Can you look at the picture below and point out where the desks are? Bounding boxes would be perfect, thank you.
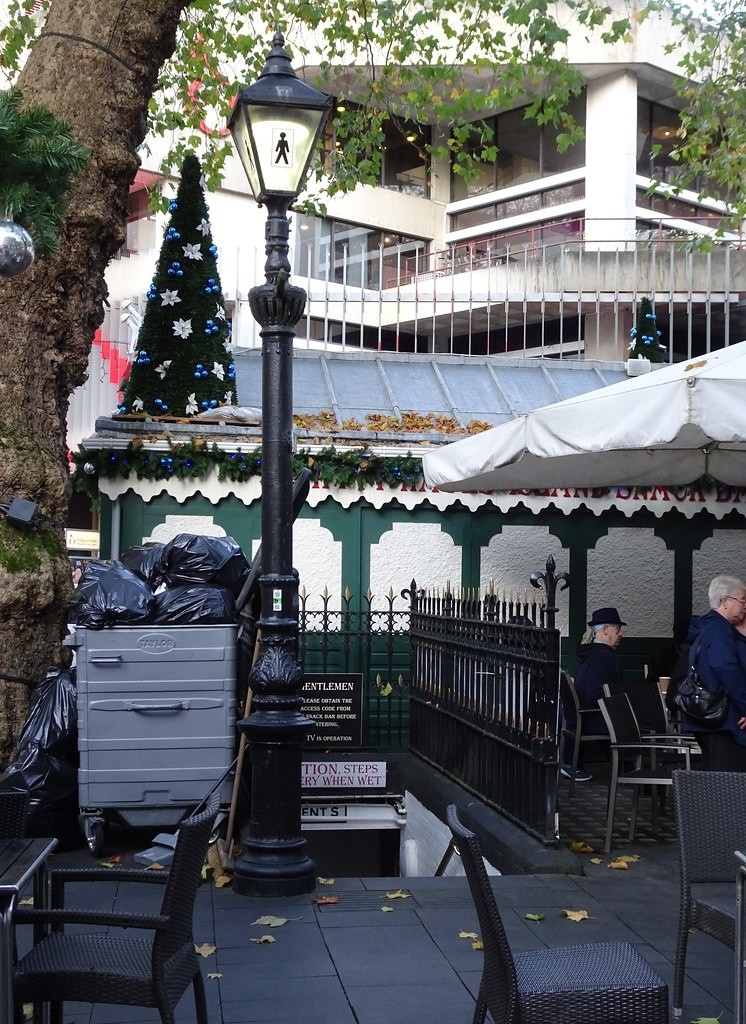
[0,838,61,1024]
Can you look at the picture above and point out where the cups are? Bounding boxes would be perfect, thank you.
[659,676,671,692]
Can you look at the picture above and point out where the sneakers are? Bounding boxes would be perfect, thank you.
[561,764,592,781]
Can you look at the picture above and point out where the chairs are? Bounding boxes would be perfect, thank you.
[672,769,746,1017]
[13,792,221,1024]
[557,668,612,798]
[597,693,692,853]
[446,804,669,1024]
[601,676,702,833]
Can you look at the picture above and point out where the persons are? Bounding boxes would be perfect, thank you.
[685,574,746,772]
[575,607,665,797]
[507,616,593,782]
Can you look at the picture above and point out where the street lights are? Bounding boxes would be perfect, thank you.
[223,30,323,898]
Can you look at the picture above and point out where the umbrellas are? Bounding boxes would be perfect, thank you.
[422,339,746,493]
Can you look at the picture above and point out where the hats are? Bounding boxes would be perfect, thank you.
[587,608,627,627]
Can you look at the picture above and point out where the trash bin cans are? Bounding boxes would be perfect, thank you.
[61,623,240,857]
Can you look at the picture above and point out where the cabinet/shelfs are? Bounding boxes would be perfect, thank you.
[66,623,238,856]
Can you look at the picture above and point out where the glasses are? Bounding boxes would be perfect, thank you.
[725,595,746,606]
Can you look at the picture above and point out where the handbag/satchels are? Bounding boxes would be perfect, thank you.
[672,666,728,727]
